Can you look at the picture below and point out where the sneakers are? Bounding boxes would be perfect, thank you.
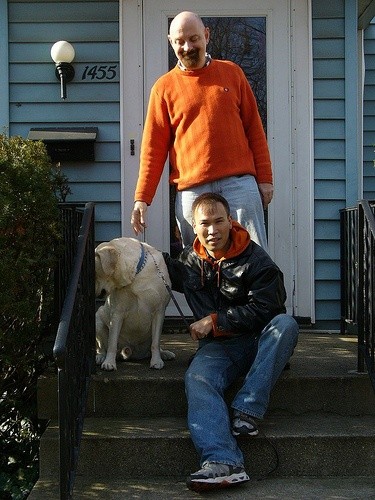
[186,460,249,491]
[232,410,258,436]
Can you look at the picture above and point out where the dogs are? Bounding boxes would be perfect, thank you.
[94,236,176,371]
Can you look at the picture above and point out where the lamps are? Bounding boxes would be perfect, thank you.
[50,40,77,101]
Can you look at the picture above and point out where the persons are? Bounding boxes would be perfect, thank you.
[131,11,274,255]
[157,193,300,494]
